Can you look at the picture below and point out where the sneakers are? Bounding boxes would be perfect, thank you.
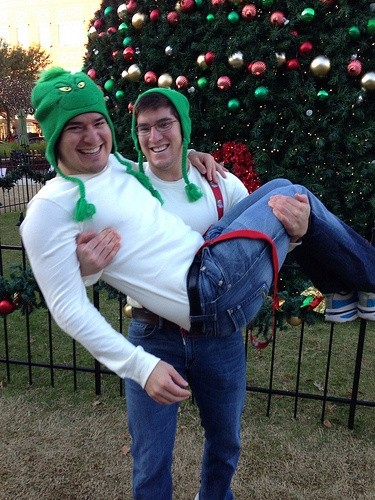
[324,291,374,324]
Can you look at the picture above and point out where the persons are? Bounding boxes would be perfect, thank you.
[76,89,312,500]
[19,66,375,405]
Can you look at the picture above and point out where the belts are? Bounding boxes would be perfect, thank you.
[131,306,175,327]
[187,248,204,335]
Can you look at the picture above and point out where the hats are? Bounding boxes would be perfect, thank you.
[30,67,163,222]
[131,87,202,203]
